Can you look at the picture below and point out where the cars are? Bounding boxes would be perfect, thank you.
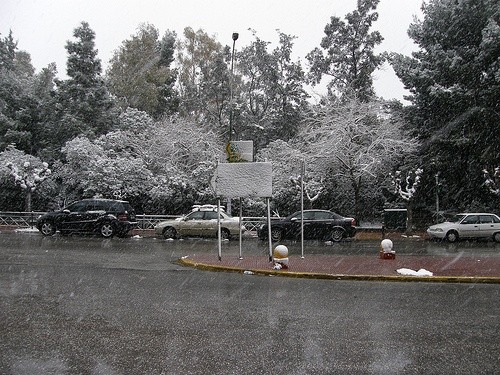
[258,209,359,243]
[154,205,246,241]
[426,213,500,243]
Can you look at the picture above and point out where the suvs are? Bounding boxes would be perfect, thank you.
[36,199,139,239]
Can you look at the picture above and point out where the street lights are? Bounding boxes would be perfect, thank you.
[227,32,240,215]
